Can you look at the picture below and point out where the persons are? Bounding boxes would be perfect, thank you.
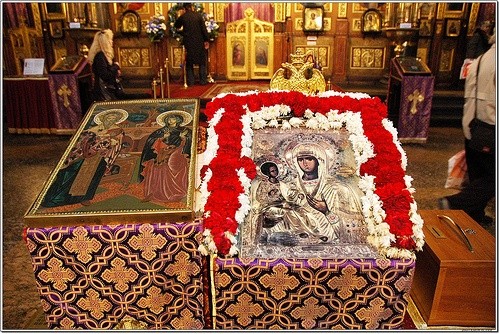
[438,21,496,222]
[173,3,208,85]
[88,28,122,102]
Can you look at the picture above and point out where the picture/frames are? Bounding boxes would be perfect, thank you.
[47,19,65,39]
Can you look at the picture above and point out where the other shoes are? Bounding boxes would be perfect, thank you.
[438,197,452,210]
[479,216,495,225]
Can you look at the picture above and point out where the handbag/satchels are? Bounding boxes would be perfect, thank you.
[444,149,470,190]
[98,77,126,103]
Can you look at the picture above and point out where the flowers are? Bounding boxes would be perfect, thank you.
[145,3,219,42]
